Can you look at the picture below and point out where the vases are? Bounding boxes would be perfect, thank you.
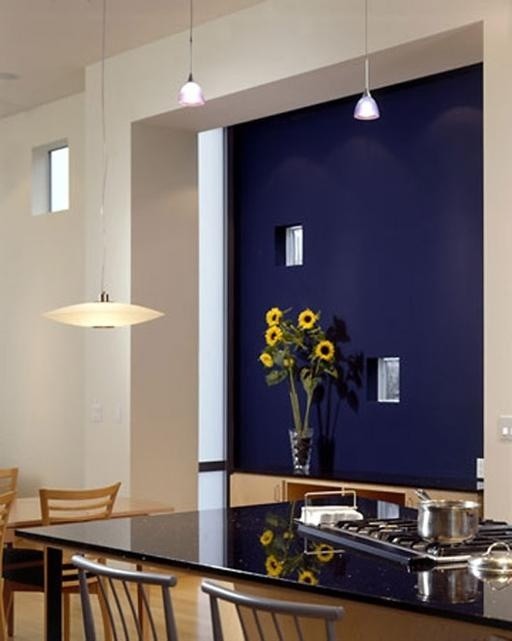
[288,429,317,474]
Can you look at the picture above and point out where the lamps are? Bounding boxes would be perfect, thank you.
[180,1,208,108]
[352,0,382,120]
[42,0,164,332]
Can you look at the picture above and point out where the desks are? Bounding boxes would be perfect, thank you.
[0,495,175,641]
[14,495,512,641]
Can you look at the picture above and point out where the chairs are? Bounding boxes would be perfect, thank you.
[0,466,18,501]
[70,554,184,640]
[200,581,345,641]
[21,483,122,640]
[1,492,22,640]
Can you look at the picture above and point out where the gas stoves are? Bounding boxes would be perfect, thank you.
[295,517,511,565]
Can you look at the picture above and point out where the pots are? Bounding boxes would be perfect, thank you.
[415,569,479,607]
[411,489,481,544]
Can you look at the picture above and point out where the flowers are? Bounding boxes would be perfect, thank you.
[260,502,338,588]
[258,306,342,428]
